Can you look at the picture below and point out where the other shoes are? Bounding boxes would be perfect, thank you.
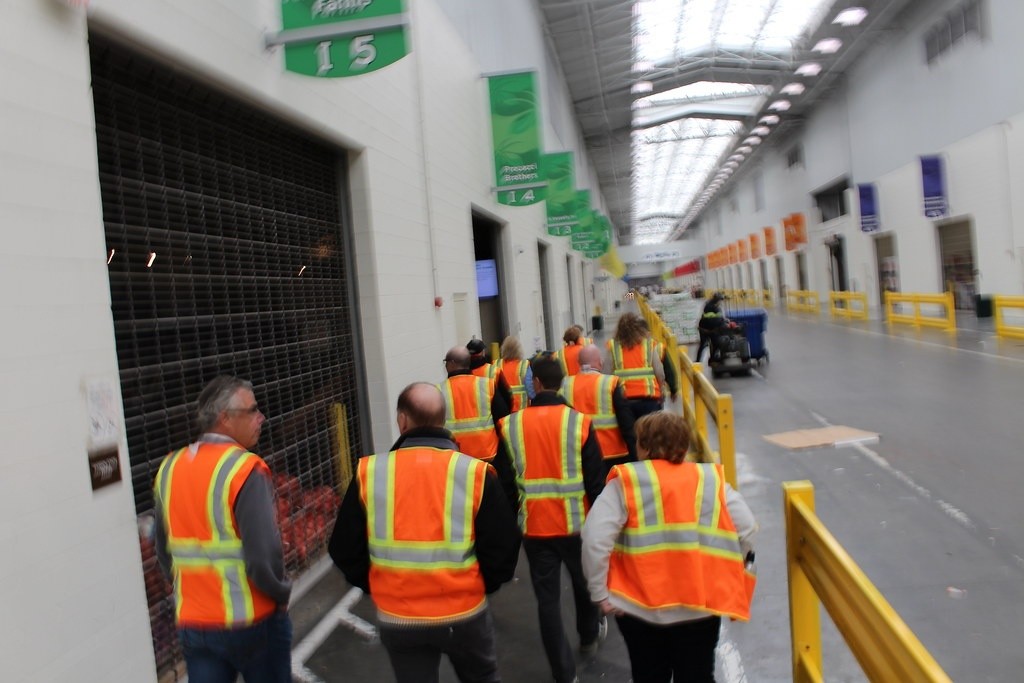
[579,616,611,657]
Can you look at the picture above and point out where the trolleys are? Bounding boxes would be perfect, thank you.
[727,310,769,366]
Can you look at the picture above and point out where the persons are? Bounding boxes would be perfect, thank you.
[490,354,608,683]
[579,409,760,683]
[326,380,524,683]
[148,372,299,683]
[693,291,728,366]
[439,307,678,601]
[636,283,664,302]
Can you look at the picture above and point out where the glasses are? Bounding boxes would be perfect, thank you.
[443,358,452,363]
[225,402,259,415]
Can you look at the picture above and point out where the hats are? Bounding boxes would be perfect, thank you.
[531,352,564,383]
[468,339,487,354]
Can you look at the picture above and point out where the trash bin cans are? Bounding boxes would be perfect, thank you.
[725,306,769,364]
